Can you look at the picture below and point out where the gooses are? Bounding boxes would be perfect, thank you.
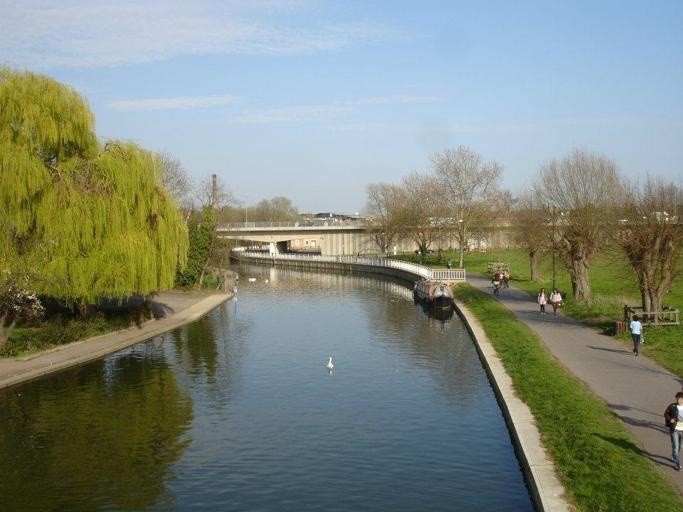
[326,356,335,370]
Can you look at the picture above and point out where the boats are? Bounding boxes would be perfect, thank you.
[414,279,454,308]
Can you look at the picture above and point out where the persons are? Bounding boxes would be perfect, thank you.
[549,288,562,315]
[447,258,452,269]
[664,392,683,471]
[492,270,510,296]
[630,315,642,356]
[537,288,548,316]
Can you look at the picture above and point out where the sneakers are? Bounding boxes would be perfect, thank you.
[675,463,682,470]
[633,349,639,356]
